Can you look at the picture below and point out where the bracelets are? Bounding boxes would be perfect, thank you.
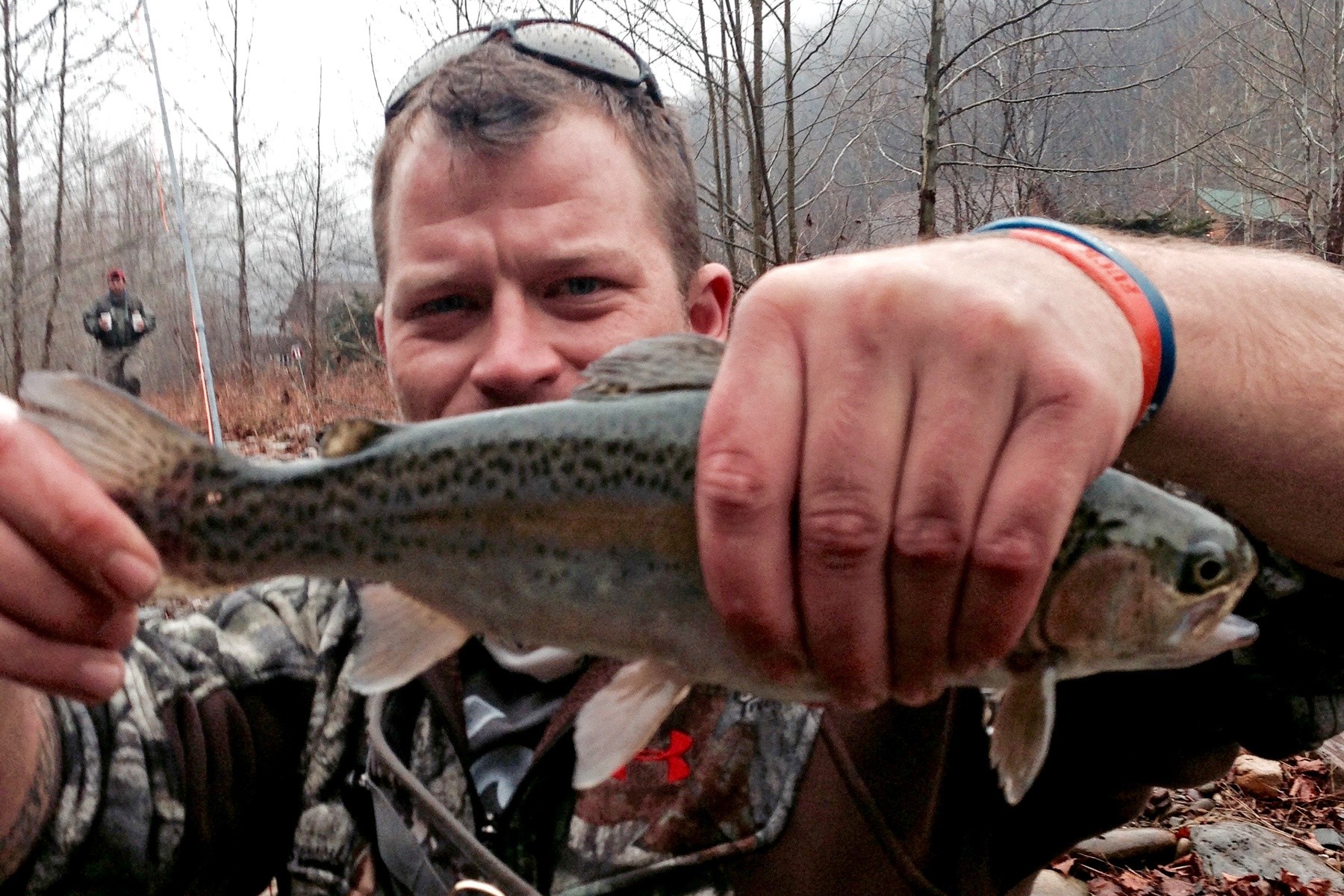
[968,217,1176,439]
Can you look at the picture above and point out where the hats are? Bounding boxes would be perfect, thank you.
[107,268,126,284]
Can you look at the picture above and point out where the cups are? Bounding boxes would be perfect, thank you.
[101,312,112,331]
[131,313,144,332]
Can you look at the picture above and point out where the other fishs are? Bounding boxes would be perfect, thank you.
[13,334,1257,807]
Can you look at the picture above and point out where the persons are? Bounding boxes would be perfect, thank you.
[0,18,1344,896]
[81,269,156,397]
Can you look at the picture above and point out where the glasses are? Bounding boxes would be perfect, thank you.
[384,19,668,128]
